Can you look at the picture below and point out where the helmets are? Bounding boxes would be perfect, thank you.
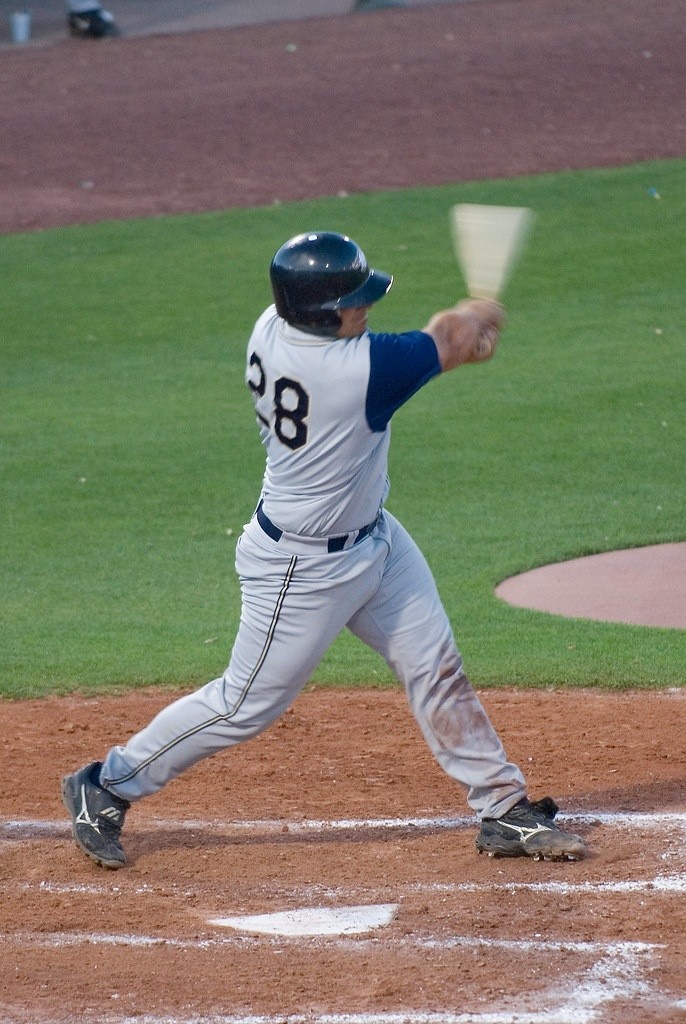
[270,231,394,336]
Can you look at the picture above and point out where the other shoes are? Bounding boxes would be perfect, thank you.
[66,8,117,39]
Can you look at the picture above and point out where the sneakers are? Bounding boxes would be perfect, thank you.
[60,761,132,870]
[475,796,587,861]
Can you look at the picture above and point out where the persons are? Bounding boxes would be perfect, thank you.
[63,231,587,870]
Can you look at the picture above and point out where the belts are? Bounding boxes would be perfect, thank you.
[256,501,379,553]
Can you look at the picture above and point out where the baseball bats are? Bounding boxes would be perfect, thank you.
[446,196,528,359]
